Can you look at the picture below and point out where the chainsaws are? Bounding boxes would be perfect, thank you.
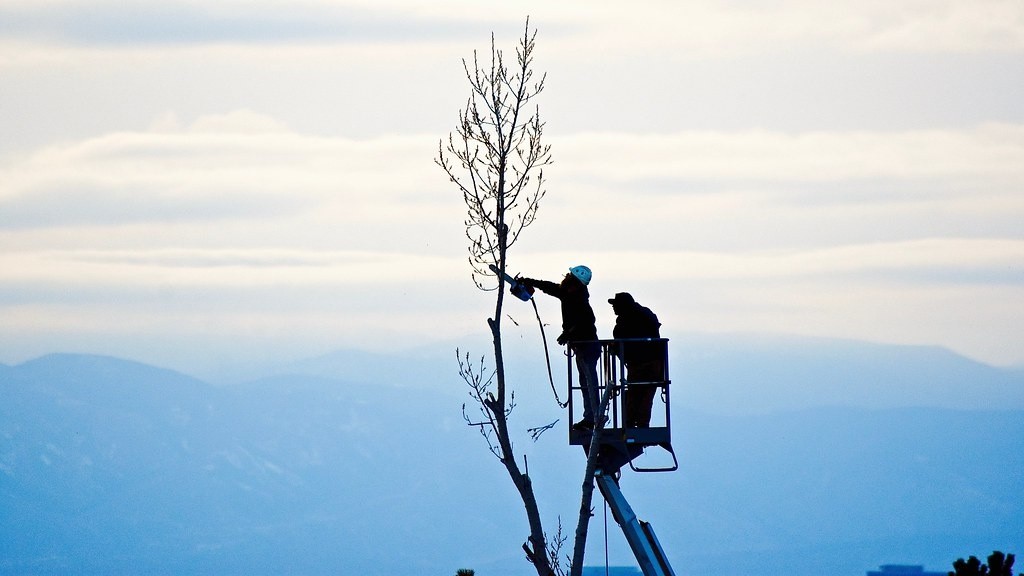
[489,263,535,301]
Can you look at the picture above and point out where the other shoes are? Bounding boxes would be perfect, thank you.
[573,414,606,431]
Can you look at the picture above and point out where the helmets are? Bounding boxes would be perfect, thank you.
[569,265,592,286]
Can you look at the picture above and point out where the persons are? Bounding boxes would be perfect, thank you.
[608,292,661,429]
[525,265,608,432]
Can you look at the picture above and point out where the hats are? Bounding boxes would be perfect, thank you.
[608,292,636,310]
